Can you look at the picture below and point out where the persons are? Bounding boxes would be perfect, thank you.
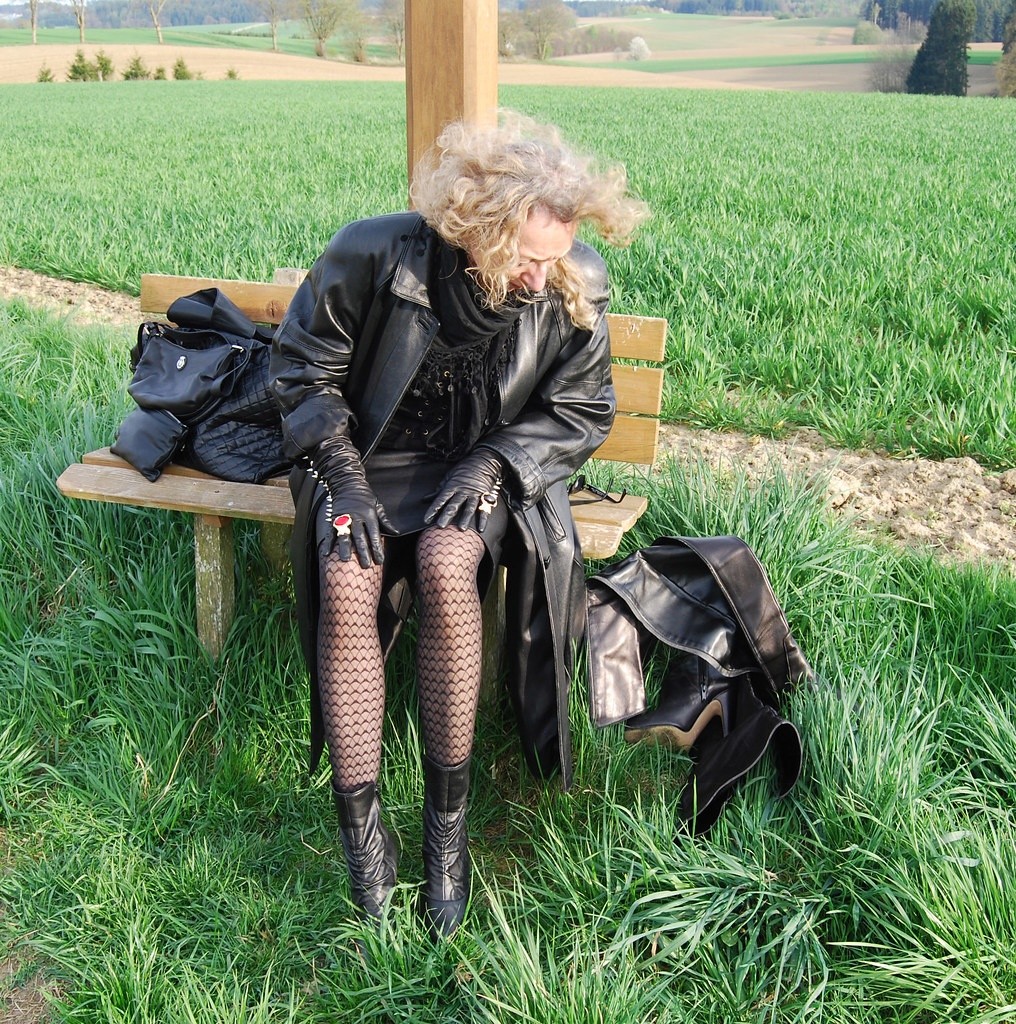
[270,117,648,941]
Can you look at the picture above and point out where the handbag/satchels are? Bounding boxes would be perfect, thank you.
[166,288,292,488]
[110,405,189,482]
[126,321,250,415]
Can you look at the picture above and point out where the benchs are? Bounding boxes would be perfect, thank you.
[54,275,668,664]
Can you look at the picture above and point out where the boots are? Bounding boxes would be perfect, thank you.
[586,536,817,846]
[422,754,470,942]
[333,781,397,969]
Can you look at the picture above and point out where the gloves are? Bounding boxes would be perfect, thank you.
[423,448,507,532]
[302,436,400,569]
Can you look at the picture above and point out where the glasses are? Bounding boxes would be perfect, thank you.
[565,475,627,507]
